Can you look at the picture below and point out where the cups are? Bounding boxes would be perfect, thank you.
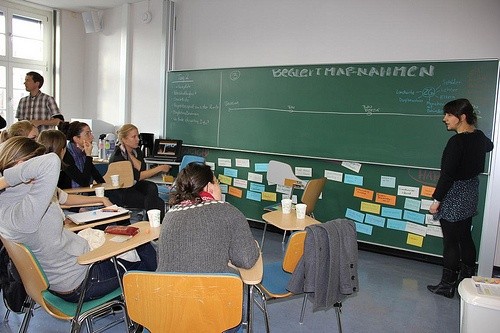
[147,209,161,228]
[110,175,119,187]
[296,203,307,219]
[281,199,292,215]
[94,187,105,197]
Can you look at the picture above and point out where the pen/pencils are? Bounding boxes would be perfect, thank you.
[102,209,118,212]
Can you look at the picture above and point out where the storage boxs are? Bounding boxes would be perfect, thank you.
[458,278,500,333]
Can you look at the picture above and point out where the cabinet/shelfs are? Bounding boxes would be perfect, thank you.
[144,158,180,184]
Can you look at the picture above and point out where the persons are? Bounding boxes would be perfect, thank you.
[426,98,494,297]
[0,71,261,333]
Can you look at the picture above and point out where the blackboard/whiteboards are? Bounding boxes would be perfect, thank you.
[163,58,500,174]
[161,143,490,263]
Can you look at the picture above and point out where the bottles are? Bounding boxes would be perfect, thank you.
[98,134,106,159]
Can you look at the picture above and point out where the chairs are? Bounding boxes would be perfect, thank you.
[0,160,343,332]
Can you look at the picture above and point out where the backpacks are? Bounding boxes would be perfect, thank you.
[0,239,28,314]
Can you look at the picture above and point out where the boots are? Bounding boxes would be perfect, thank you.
[427,267,459,298]
[454,262,474,289]
